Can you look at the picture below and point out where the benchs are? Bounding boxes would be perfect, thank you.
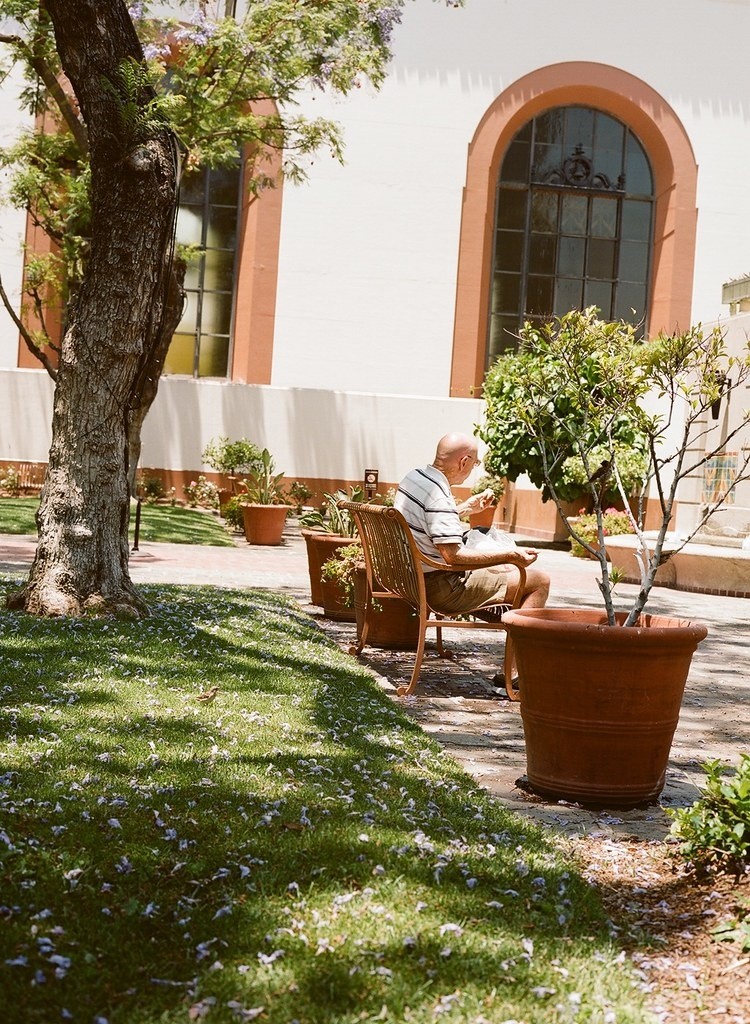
[335,498,526,702]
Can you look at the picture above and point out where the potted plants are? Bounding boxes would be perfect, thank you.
[298,485,394,623]
[200,436,263,518]
[319,538,430,650]
[469,304,750,811]
[469,476,505,529]
[226,449,294,546]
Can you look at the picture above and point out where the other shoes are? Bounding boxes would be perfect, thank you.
[491,670,520,696]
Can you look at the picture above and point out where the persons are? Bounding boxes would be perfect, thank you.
[396,432,550,697]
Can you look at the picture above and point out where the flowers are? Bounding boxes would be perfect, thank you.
[568,508,636,556]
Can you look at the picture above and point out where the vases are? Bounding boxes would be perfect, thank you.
[566,516,590,558]
[588,530,610,562]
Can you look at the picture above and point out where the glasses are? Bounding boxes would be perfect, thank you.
[461,455,481,466]
